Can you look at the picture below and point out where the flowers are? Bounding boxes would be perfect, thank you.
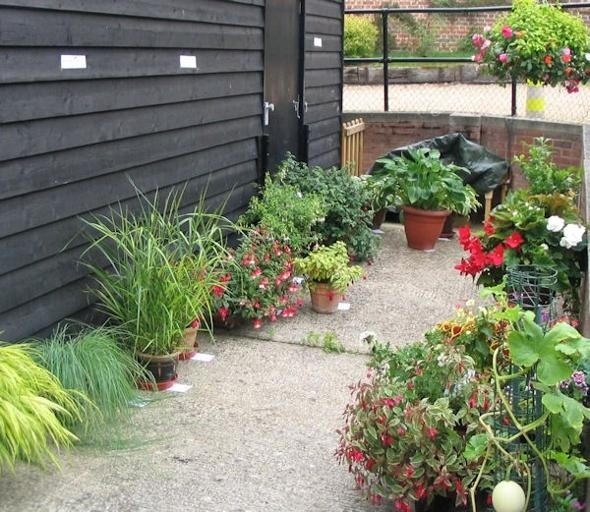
[291,241,367,301]
[455,189,588,287]
[470,1,589,94]
[211,222,304,330]
[334,334,512,512]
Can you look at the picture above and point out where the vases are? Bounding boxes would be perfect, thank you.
[525,77,546,121]
[308,280,344,314]
[415,473,456,511]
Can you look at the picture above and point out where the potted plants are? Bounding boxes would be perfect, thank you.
[357,149,482,249]
